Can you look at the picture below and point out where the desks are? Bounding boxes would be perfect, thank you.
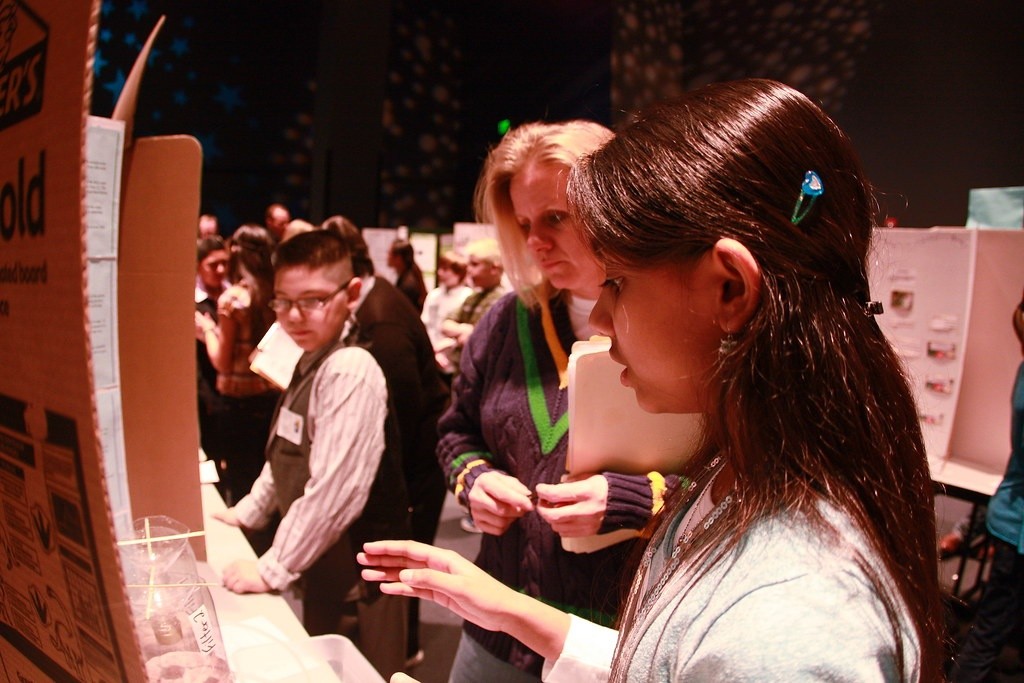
[198,447,336,683]
[926,457,1004,616]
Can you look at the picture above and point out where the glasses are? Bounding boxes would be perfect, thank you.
[267,278,350,313]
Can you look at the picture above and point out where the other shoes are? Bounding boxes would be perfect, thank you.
[935,532,962,556]
[977,544,995,561]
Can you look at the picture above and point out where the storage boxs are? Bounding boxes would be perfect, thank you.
[309,632,386,682]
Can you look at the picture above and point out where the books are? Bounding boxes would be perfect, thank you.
[561,334,702,555]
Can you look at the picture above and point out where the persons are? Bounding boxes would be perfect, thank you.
[195,202,506,506]
[210,231,408,682]
[938,292,1023,683]
[436,120,703,683]
[353,79,961,683]
[341,234,446,668]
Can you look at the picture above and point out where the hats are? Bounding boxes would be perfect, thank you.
[442,251,466,265]
[461,238,504,270]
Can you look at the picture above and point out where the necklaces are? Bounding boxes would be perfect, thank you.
[678,464,726,546]
